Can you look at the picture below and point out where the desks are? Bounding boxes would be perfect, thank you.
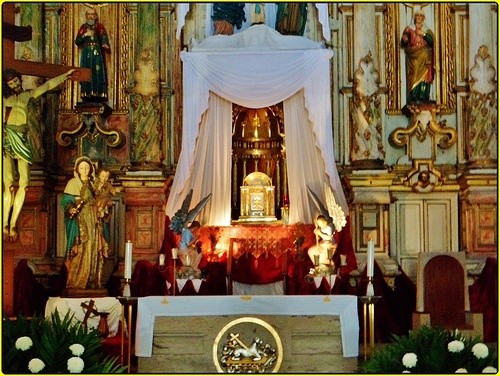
[304,274,341,296]
[45,296,127,338]
[134,295,359,373]
[361,296,382,351]
[176,278,208,296]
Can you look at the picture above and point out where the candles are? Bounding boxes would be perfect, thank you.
[124,240,132,279]
[159,254,165,265]
[227,239,233,272]
[341,254,346,265]
[328,250,332,260]
[367,238,374,277]
[281,238,287,273]
[185,255,191,267]
[314,255,319,265]
[172,248,178,259]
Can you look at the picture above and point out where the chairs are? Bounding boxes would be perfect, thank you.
[412,251,483,341]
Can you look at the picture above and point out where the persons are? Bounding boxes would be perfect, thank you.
[3,69,74,242]
[400,11,437,102]
[178,220,203,273]
[94,168,119,213]
[61,156,113,290]
[75,9,110,104]
[307,215,336,270]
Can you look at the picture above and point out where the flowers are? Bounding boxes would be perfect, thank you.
[2,306,128,374]
[362,324,498,374]
